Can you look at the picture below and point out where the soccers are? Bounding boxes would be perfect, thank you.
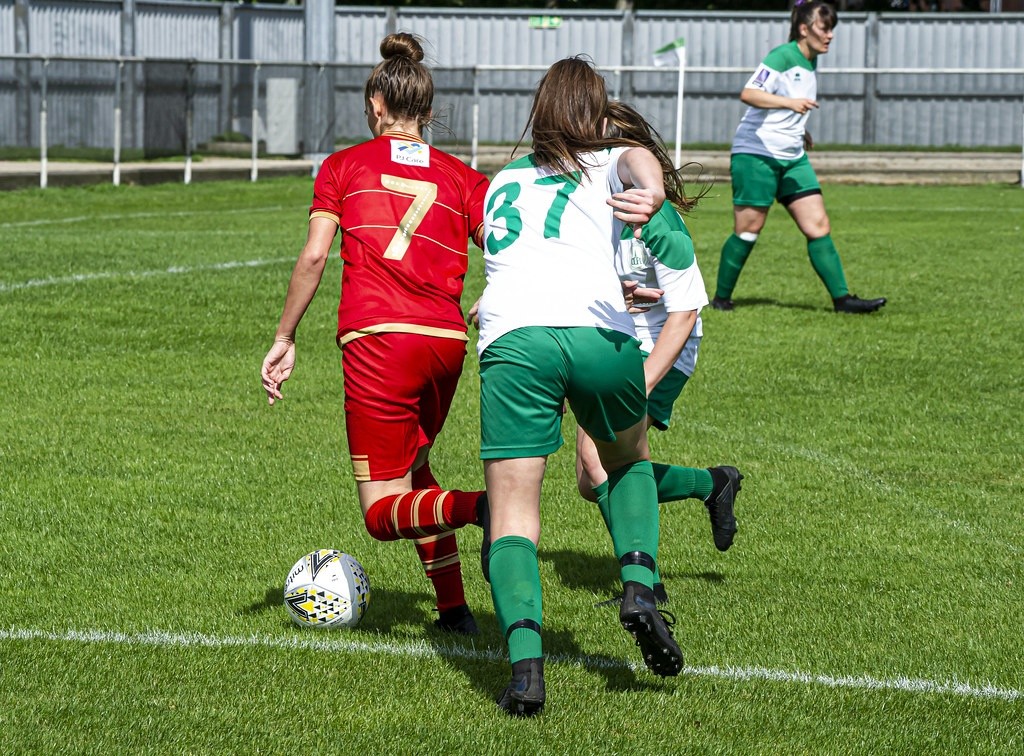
[283,547,372,633]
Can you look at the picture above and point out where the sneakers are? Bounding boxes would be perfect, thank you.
[619,583,685,678]
[482,492,492,584]
[602,583,669,605]
[834,295,888,314]
[496,660,546,718]
[707,466,745,552]
[712,301,736,312]
[431,609,478,635]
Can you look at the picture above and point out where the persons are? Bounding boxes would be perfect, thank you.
[476,53,685,717]
[261,33,492,632]
[709,0,888,312]
[563,103,744,607]
